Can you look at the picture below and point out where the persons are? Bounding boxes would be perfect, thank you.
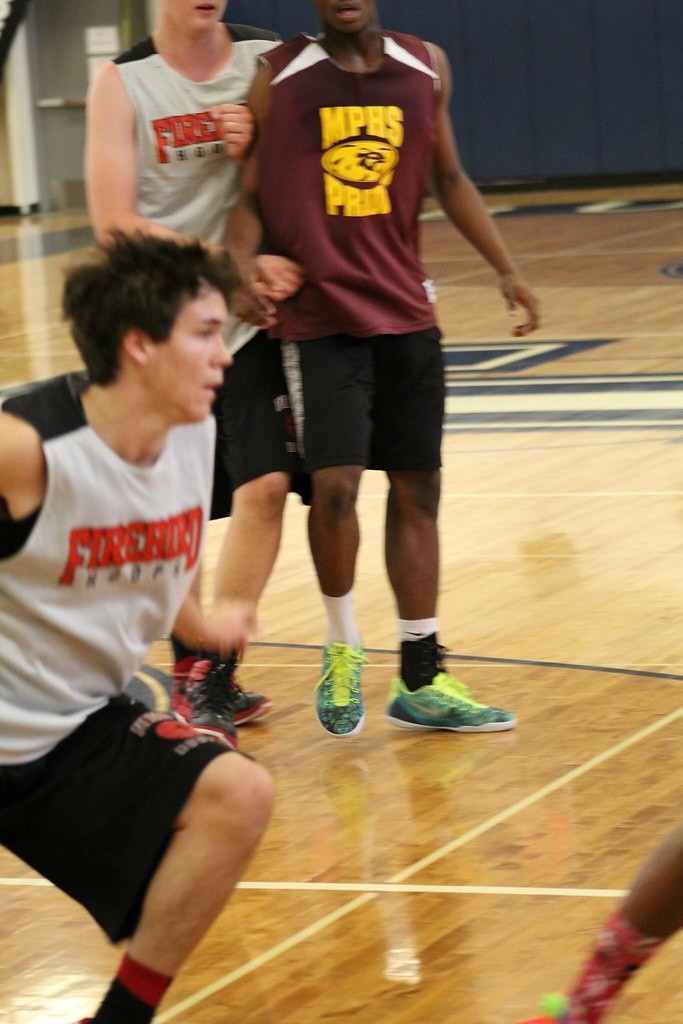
[224,1,541,737]
[0,229,272,1024]
[521,823,683,1024]
[84,1,300,732]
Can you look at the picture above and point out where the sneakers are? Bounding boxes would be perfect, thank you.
[315,634,367,738]
[318,752,378,847]
[387,729,518,793]
[168,635,274,749]
[385,670,518,734]
